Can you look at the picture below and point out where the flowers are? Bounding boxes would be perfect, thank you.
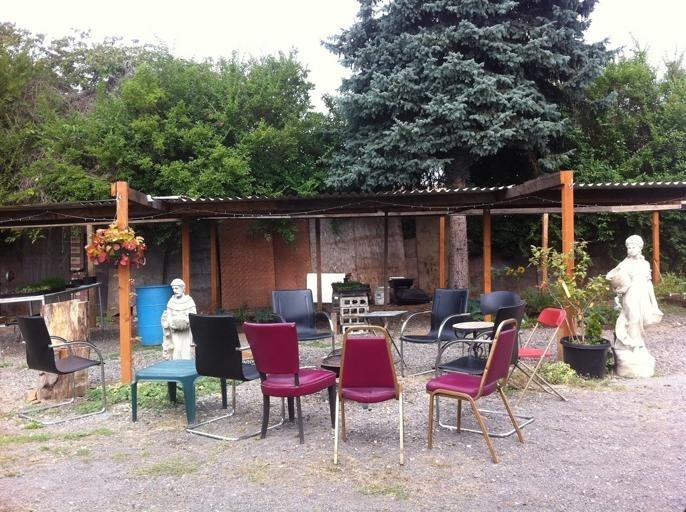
[84,225,149,269]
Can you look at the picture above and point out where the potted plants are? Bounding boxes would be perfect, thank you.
[529,239,612,381]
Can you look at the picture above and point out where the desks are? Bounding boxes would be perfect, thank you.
[451,321,495,362]
[356,311,408,371]
[1,282,103,328]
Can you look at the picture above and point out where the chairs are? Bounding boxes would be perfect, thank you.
[425,317,525,464]
[399,288,470,377]
[271,288,335,355]
[461,290,523,389]
[331,325,406,467]
[436,300,535,437]
[188,312,285,442]
[242,321,336,445]
[16,315,105,427]
[517,306,568,402]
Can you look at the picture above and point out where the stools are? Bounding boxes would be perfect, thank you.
[130,359,227,425]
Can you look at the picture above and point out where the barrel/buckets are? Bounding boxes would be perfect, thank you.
[135,284,174,346]
[374,286,390,305]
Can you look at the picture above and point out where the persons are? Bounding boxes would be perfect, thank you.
[605,234,664,377]
[160,278,198,361]
